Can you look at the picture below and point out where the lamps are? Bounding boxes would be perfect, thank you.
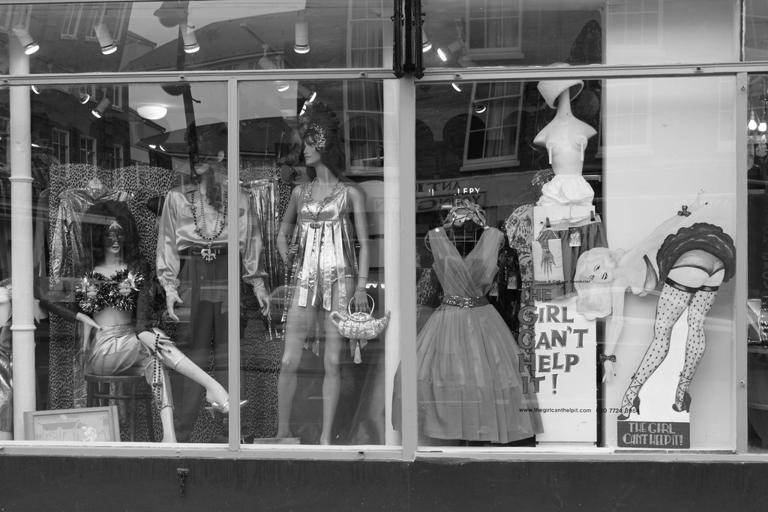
[93,24,118,55]
[11,23,40,55]
[421,29,486,114]
[181,24,200,54]
[31,84,167,120]
[239,22,317,103]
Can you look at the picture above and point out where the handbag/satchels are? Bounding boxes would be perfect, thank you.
[330,294,392,340]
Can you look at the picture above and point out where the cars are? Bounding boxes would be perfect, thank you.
[162,285,247,344]
[530,287,735,363]
[262,285,298,330]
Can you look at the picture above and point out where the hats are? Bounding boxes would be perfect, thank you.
[536,61,583,97]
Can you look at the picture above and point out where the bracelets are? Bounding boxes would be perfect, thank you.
[597,352,616,364]
[674,205,692,218]
[356,275,368,279]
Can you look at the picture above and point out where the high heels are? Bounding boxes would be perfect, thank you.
[205,398,248,418]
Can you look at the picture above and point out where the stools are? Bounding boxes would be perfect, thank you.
[85,371,154,441]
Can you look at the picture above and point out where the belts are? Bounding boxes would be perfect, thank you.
[189,247,226,256]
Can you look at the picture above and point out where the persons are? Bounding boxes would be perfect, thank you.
[154,115,270,440]
[40,199,248,442]
[407,200,544,446]
[573,185,737,422]
[530,79,599,221]
[268,109,371,444]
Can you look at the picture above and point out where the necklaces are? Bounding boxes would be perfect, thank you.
[305,176,339,230]
[190,181,225,240]
